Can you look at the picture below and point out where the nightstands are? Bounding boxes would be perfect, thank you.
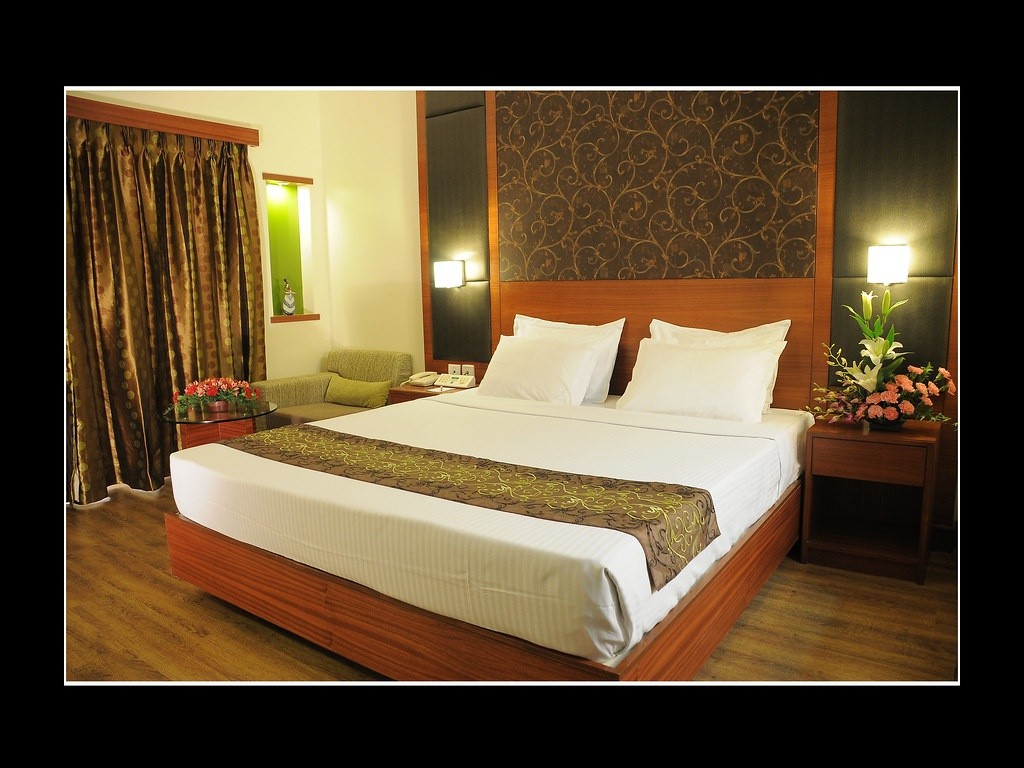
[390,376,480,405]
[799,416,941,584]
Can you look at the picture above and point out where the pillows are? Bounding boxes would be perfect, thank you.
[327,371,391,409]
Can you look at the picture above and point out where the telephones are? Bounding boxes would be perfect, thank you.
[408,371,438,387]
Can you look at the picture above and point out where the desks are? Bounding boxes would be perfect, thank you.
[157,396,277,452]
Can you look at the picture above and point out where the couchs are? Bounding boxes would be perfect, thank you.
[249,347,414,433]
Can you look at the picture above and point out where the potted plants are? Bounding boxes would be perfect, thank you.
[206,398,228,413]
[865,407,907,432]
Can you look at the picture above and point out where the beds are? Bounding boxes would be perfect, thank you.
[162,272,823,679]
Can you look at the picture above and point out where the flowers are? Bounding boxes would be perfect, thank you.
[808,288,957,433]
[169,376,259,413]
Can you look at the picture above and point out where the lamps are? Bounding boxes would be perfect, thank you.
[432,260,468,291]
[267,184,289,206]
[864,239,918,289]
